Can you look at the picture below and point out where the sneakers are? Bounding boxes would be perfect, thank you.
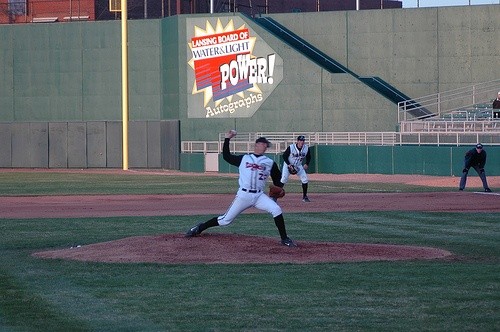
[187,222,205,237]
[281,238,298,247]
[302,197,310,202]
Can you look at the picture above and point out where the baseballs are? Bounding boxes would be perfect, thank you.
[230,129,237,136]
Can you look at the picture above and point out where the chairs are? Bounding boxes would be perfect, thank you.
[440,104,500,128]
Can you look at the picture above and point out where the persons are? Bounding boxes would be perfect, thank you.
[185,129,299,249]
[491,91,500,119]
[459,142,491,192]
[280,136,312,203]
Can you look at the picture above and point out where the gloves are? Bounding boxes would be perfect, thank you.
[480,169,484,172]
[463,168,468,174]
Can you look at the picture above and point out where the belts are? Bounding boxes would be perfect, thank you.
[242,189,260,193]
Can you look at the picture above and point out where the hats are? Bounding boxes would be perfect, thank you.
[297,136,305,141]
[256,137,271,147]
[476,144,483,149]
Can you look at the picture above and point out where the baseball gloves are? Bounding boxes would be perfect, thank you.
[268,184,286,199]
[288,165,299,175]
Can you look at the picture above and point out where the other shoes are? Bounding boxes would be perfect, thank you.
[485,188,491,192]
[459,188,463,191]
